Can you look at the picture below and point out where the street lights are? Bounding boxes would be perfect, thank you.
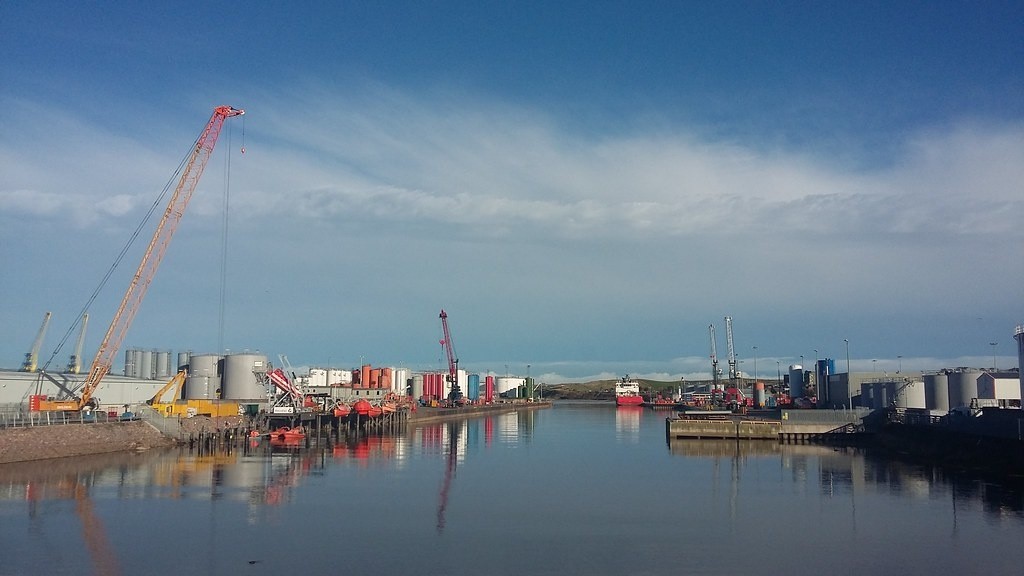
[738,360,745,394]
[800,354,805,383]
[751,346,759,383]
[813,348,820,401]
[990,341,999,374]
[872,359,878,372]
[777,360,781,401]
[897,356,904,378]
[844,338,852,411]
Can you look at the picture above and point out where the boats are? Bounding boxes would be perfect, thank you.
[616,375,644,407]
[250,427,305,449]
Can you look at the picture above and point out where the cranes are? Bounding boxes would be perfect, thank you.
[65,312,89,375]
[22,309,51,373]
[438,307,469,408]
[20,104,245,426]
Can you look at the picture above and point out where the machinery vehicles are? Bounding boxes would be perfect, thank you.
[254,354,417,418]
[141,365,240,422]
